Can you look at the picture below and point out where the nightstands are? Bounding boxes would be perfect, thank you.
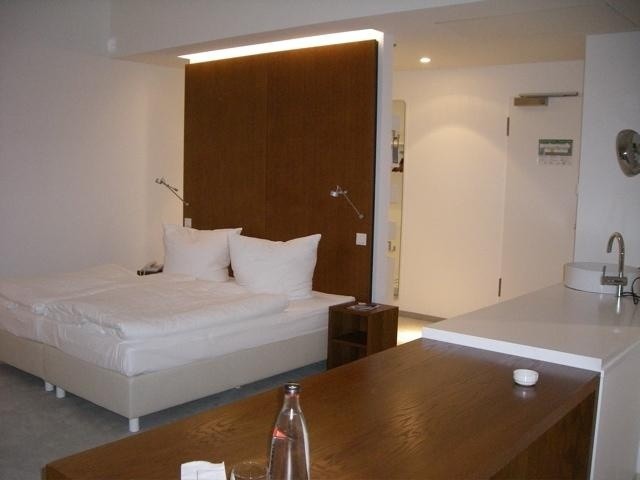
[327,300,399,370]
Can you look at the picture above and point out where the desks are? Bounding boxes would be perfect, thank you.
[41,337,601,480]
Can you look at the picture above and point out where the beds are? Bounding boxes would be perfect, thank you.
[0,265,356,432]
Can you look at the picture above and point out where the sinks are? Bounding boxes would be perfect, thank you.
[563,261,640,295]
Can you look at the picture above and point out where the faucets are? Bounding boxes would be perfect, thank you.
[601,232,627,297]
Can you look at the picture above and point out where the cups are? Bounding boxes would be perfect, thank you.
[229,460,269,479]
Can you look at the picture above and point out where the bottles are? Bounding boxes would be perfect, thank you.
[269,383,310,480]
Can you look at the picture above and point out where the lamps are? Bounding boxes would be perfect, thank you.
[156,178,189,207]
[330,186,364,220]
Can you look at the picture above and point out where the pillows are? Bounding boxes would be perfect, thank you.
[228,234,322,298]
[163,223,243,283]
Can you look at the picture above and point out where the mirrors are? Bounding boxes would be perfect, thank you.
[392,99,406,299]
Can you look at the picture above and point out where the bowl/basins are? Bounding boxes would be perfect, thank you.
[514,367,540,388]
